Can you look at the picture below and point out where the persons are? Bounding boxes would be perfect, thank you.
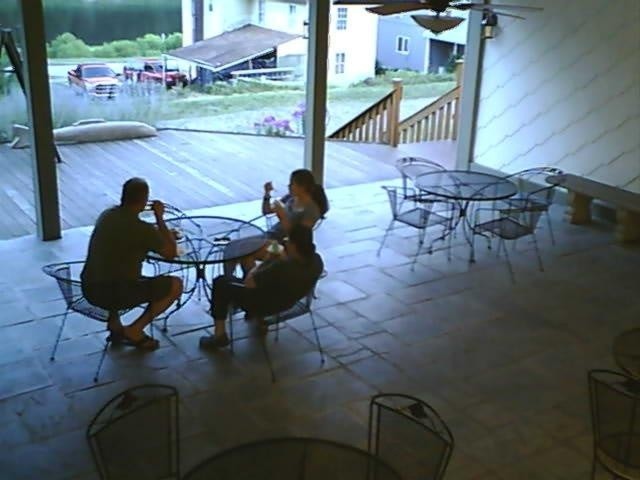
[224,169,329,277]
[200,224,323,349]
[80,176,184,349]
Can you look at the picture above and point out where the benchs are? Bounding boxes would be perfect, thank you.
[545,173,640,243]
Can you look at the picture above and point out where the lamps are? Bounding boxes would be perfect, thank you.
[410,10,465,35]
[482,13,496,38]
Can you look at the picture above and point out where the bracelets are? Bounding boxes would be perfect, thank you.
[264,195,271,201]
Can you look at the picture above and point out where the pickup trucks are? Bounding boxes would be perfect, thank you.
[65,61,124,102]
[123,58,186,90]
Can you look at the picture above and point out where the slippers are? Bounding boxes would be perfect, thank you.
[120,335,160,351]
[105,331,122,342]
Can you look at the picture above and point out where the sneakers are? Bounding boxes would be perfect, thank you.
[199,333,229,349]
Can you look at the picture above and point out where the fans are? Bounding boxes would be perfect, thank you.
[332,0,544,21]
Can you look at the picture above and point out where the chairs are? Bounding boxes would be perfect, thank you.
[478,166,563,247]
[587,368,640,480]
[138,200,203,320]
[86,384,180,480]
[376,186,454,271]
[205,208,281,288]
[470,182,560,281]
[223,271,325,382]
[41,260,177,382]
[395,156,463,245]
[367,393,454,480]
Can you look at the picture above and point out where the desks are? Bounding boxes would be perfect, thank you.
[613,327,640,384]
